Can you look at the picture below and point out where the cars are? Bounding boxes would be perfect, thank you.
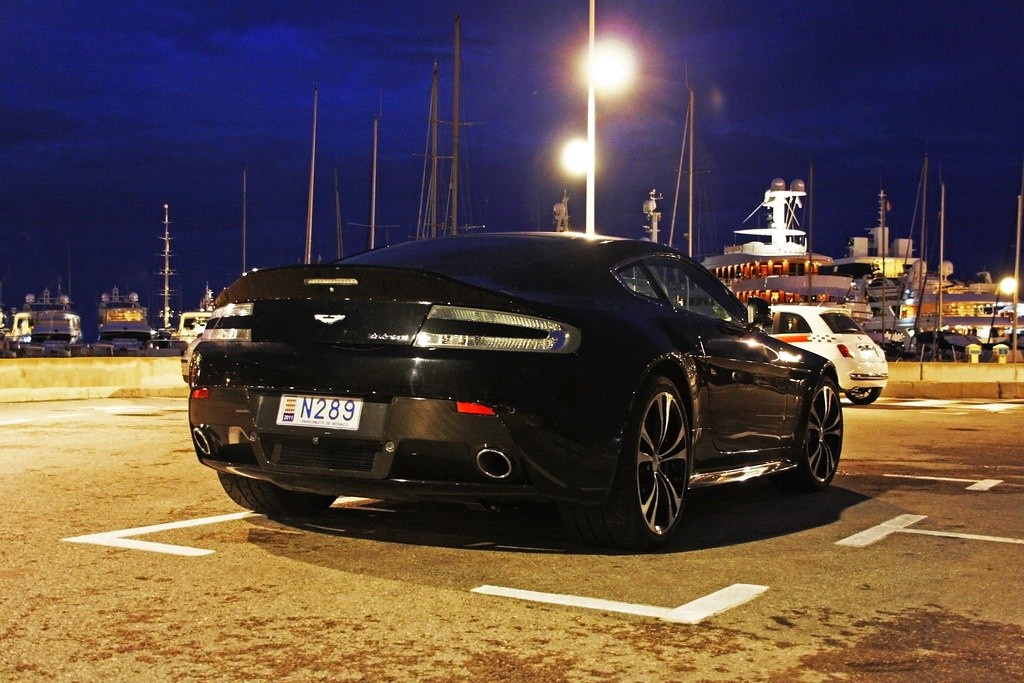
[718,304,888,404]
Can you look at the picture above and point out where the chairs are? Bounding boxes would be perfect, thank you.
[797,317,812,333]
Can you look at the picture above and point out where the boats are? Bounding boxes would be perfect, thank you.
[172,281,221,349]
[699,161,874,348]
[829,155,1023,363]
[3,288,84,355]
[95,286,154,349]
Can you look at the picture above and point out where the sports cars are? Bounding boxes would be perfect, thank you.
[186,229,843,547]
